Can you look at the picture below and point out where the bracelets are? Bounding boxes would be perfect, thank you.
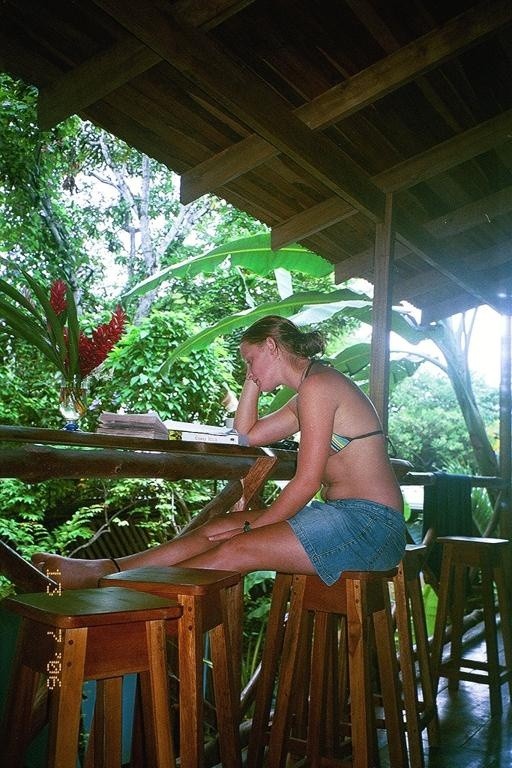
[242,520,253,532]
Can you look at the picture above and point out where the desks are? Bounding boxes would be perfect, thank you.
[1,425,412,482]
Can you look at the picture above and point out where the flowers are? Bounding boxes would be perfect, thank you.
[0,270,129,412]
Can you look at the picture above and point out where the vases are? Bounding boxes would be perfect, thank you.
[58,379,87,431]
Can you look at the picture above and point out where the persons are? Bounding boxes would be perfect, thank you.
[30,314,407,589]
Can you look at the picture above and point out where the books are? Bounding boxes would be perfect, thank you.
[181,432,250,447]
[163,419,238,436]
[95,409,170,440]
